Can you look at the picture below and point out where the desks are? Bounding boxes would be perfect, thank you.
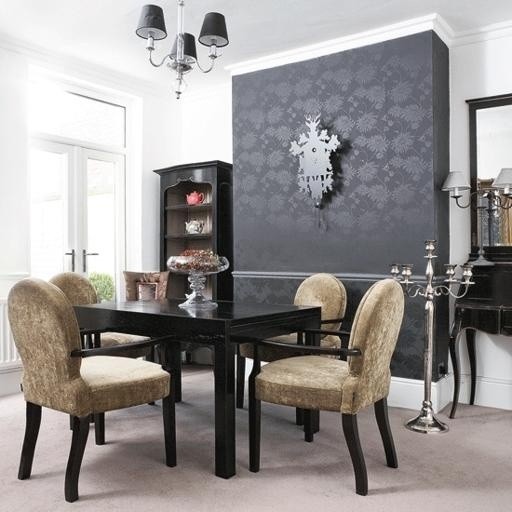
[450,302,512,421]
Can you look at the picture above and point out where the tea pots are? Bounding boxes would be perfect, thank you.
[185,220,205,234]
[186,190,204,206]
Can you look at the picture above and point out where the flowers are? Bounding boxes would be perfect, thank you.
[176,248,217,272]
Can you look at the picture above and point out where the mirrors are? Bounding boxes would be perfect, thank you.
[466,94,512,255]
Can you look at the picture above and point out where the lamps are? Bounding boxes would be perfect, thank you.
[489,165,512,208]
[136,0,229,100]
[439,172,481,208]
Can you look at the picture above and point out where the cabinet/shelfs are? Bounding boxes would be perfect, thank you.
[152,160,234,306]
